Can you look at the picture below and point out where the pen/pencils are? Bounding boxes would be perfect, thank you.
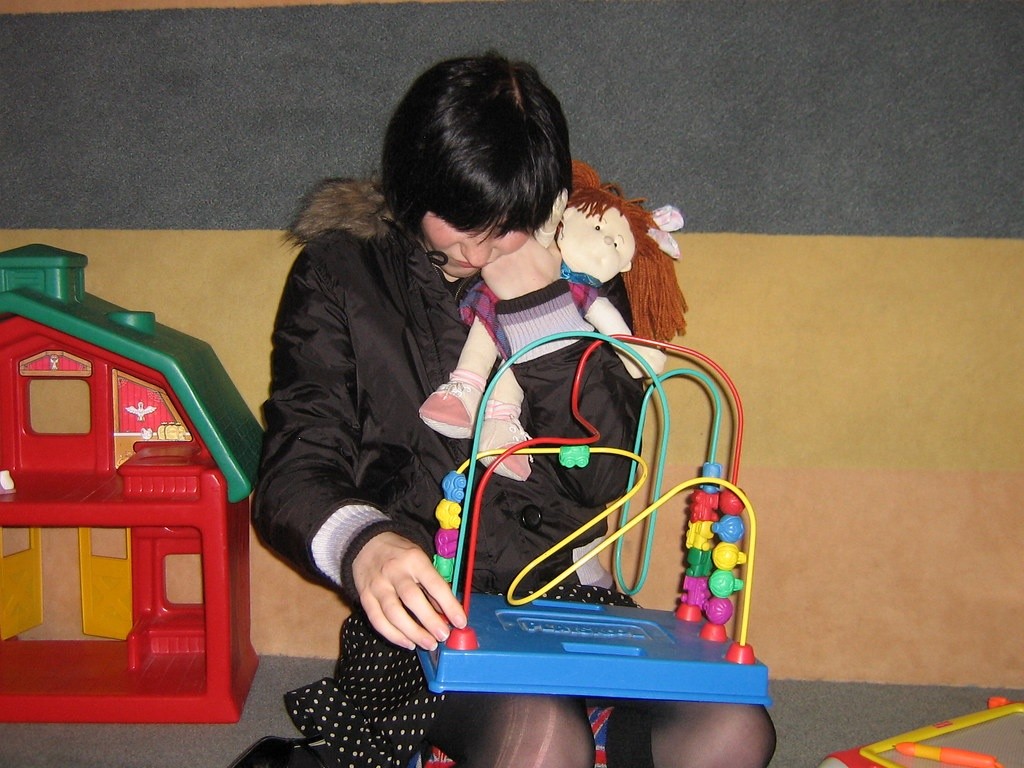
[892,741,1005,768]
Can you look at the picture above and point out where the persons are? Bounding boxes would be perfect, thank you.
[419,160,688,481]
[251,47,777,768]
